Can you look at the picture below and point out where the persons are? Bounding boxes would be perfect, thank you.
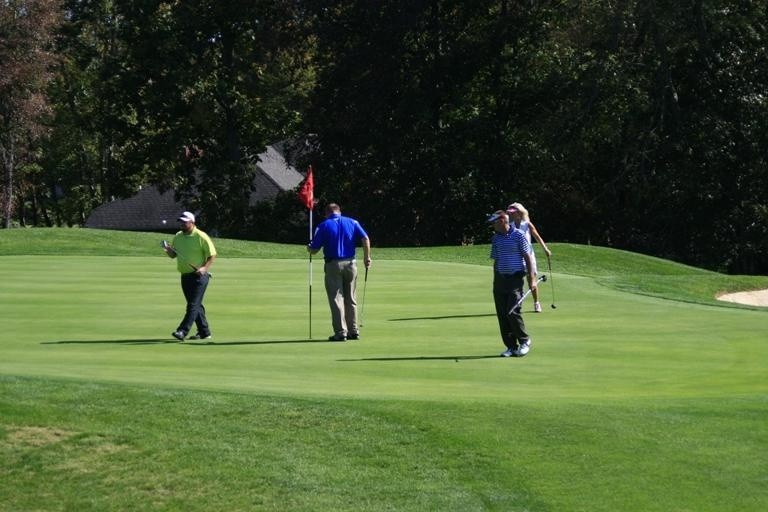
[505,201,553,314]
[307,202,372,342]
[163,210,218,343]
[487,209,538,359]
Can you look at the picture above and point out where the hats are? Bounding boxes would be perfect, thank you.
[487,209,510,224]
[506,207,518,214]
[176,210,195,224]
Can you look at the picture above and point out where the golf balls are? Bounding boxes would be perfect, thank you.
[162,220,167,224]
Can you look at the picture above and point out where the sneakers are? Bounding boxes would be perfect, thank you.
[533,301,542,313]
[328,333,348,343]
[500,346,519,357]
[171,330,187,342]
[189,332,212,340]
[347,332,361,341]
[516,336,532,357]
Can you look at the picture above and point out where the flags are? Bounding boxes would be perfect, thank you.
[296,166,316,210]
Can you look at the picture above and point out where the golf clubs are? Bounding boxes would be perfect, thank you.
[508,275,547,316]
[161,241,198,271]
[360,261,370,327]
[547,254,556,308]
